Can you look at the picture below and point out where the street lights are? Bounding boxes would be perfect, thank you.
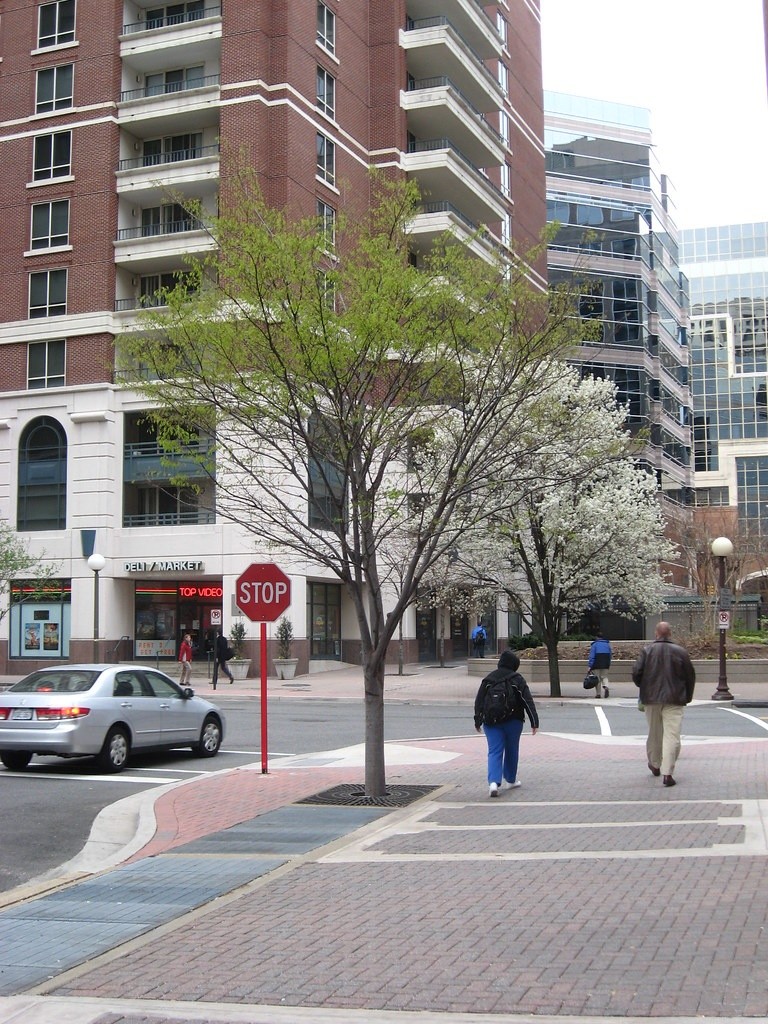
[87,553,106,665]
[711,536,732,701]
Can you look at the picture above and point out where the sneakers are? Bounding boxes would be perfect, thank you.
[489,782,498,797]
[506,781,522,790]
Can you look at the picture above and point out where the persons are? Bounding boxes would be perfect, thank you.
[587,631,612,698]
[472,652,541,799]
[632,621,696,785]
[208,629,235,685]
[471,621,488,658]
[178,633,193,686]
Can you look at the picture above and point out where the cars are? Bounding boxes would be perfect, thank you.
[0,662,228,774]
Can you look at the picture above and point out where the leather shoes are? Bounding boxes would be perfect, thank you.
[647,762,660,776]
[663,775,676,785]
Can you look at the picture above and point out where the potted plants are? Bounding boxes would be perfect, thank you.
[220,611,254,681]
[272,615,299,681]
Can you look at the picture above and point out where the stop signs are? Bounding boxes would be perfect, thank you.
[236,563,291,623]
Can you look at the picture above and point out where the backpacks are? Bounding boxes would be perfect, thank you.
[482,671,521,726]
[476,628,485,646]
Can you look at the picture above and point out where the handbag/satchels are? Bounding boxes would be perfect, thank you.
[225,647,233,661]
[583,668,598,690]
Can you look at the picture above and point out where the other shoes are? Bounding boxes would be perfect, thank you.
[595,695,601,698]
[209,682,217,684]
[605,689,609,698]
[230,677,234,684]
